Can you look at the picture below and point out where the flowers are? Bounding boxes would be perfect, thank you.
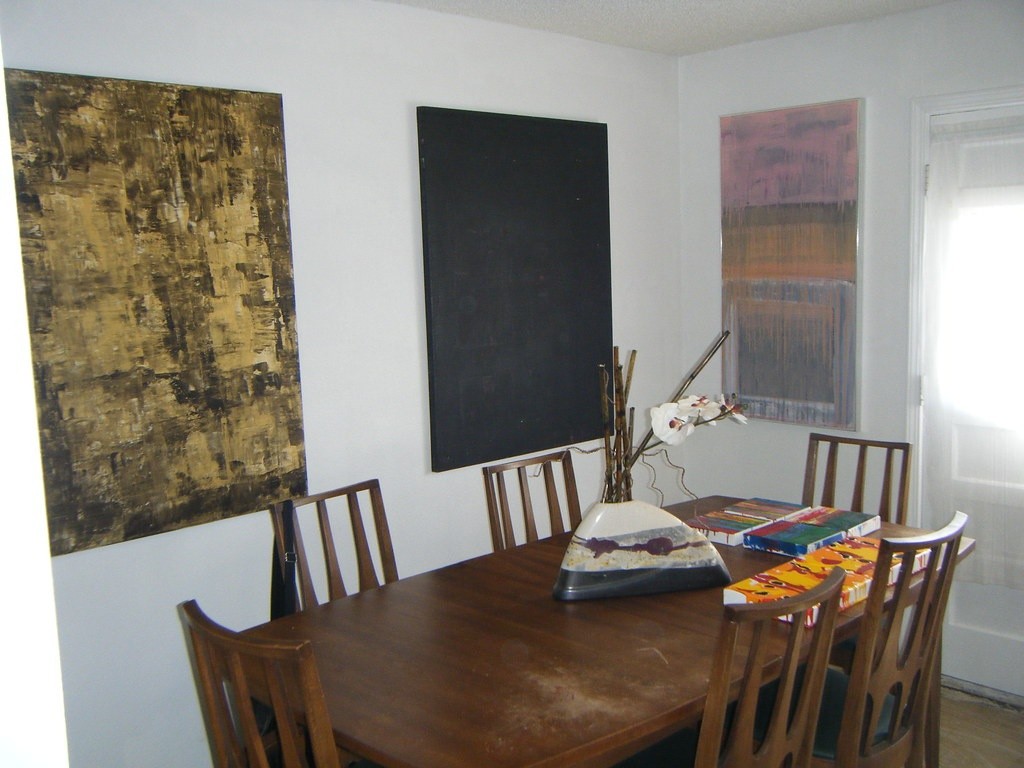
[529,395,745,531]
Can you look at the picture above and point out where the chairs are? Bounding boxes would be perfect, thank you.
[617,511,968,768]
[270,477,401,615]
[176,599,372,768]
[803,432,913,527]
[484,450,583,552]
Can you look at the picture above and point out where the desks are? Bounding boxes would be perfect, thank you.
[213,495,978,768]
